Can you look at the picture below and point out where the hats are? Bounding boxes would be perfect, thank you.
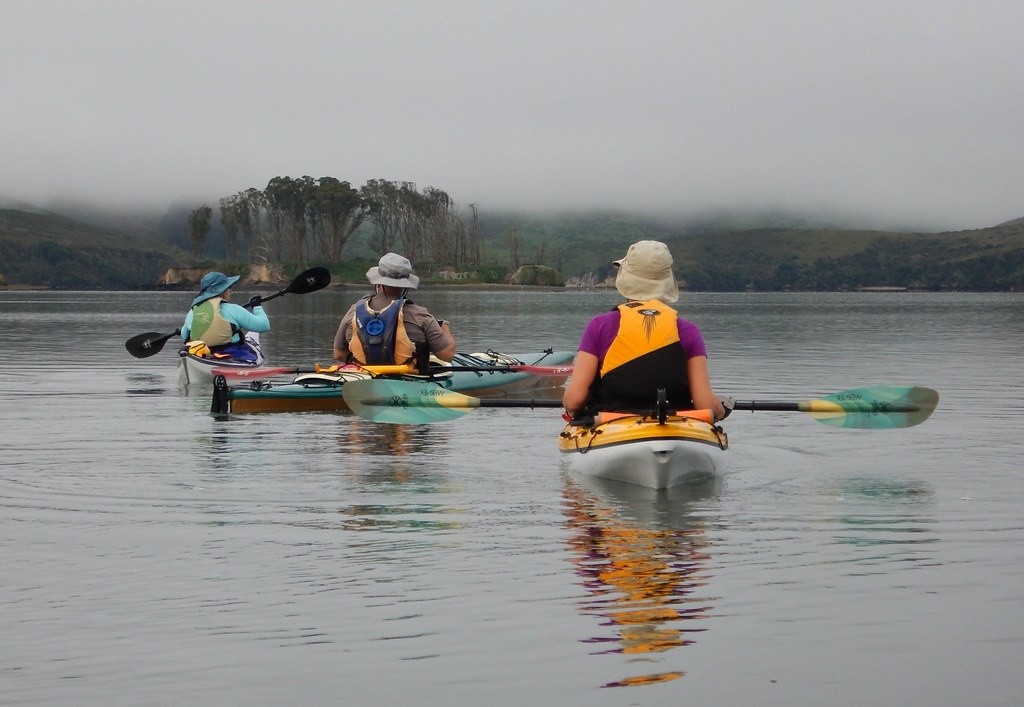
[612,238,680,304]
[191,270,239,309]
[366,252,420,289]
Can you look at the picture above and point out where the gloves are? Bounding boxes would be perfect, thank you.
[713,394,738,421]
[249,295,262,308]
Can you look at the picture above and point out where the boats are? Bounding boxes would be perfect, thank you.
[560,414,730,496]
[178,340,266,386]
[209,346,575,415]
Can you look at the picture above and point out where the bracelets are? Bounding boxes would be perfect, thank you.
[561,409,574,423]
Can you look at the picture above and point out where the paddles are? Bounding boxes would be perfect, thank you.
[337,378,942,432]
[125,266,336,359]
[212,363,577,378]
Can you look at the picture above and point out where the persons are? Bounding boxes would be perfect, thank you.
[563,240,726,423]
[181,272,270,362]
[333,253,456,379]
